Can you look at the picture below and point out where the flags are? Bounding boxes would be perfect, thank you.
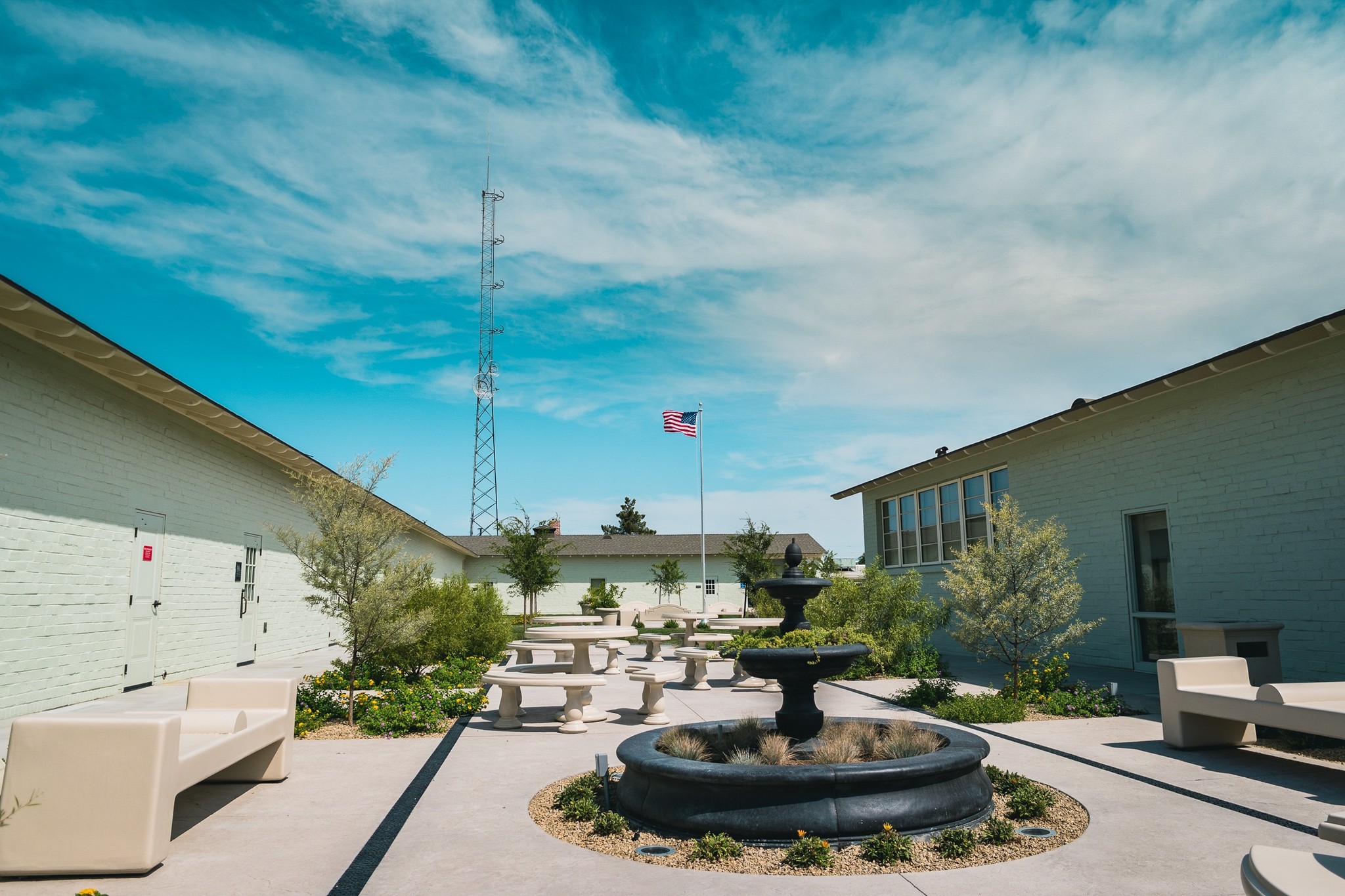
[662,410,699,437]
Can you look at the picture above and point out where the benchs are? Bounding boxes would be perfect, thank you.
[1156,655,1345,750]
[625,663,682,725]
[0,677,298,879]
[674,646,720,690]
[481,662,606,734]
[595,639,631,674]
[506,639,574,664]
[637,633,671,662]
[671,630,733,647]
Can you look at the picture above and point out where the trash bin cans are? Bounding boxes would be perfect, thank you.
[1180,620,1285,687]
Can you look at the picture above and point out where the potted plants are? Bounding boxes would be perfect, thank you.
[578,582,627,625]
[612,715,995,849]
[720,626,871,684]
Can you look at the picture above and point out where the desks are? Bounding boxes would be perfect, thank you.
[533,616,603,644]
[706,618,785,687]
[524,626,639,673]
[661,614,718,660]
